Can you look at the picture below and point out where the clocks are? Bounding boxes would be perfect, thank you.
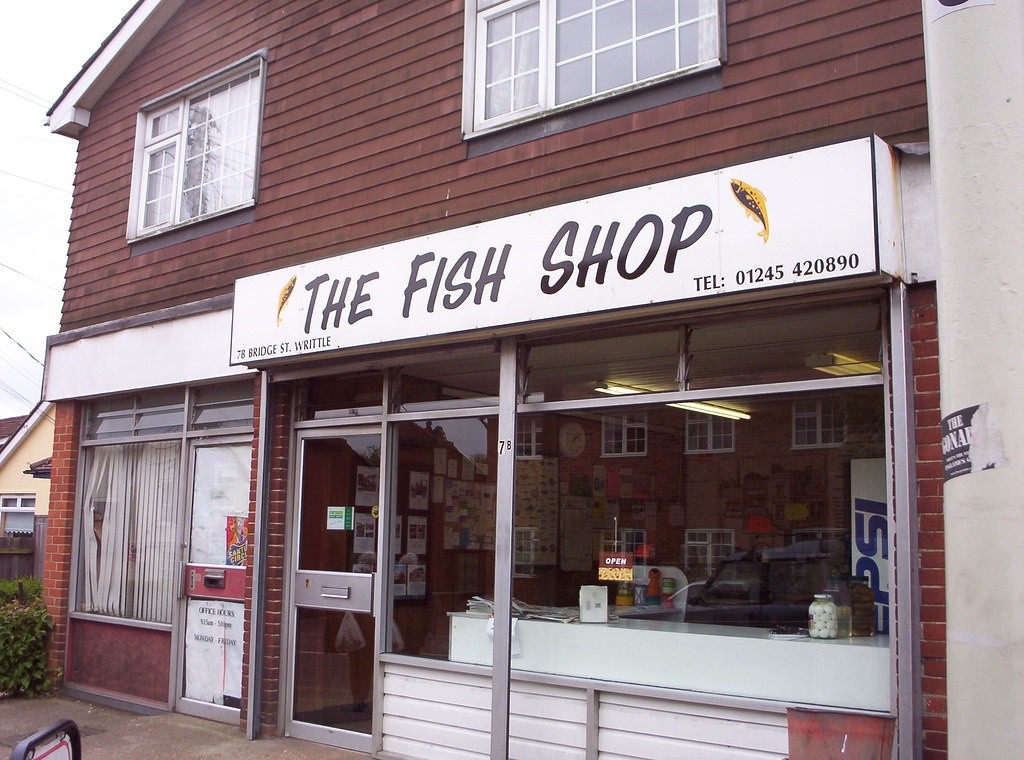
[558,422,585,457]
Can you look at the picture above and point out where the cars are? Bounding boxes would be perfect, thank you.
[655,533,853,631]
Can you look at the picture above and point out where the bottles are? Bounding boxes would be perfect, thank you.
[847,583,876,637]
[822,569,852,637]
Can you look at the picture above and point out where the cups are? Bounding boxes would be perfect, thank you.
[662,578,675,610]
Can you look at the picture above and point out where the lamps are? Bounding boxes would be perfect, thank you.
[594,380,753,421]
[804,352,880,376]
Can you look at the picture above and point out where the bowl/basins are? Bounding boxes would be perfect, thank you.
[818,538,848,553]
